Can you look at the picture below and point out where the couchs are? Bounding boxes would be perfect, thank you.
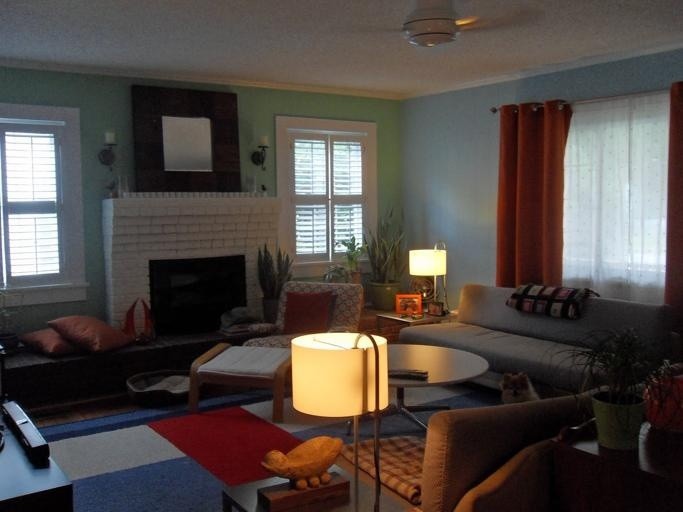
[398,283,671,395]
[334,384,614,512]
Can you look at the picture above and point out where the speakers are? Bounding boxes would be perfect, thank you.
[2,400,50,460]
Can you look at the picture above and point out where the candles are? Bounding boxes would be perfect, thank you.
[259,135,267,145]
[105,128,116,143]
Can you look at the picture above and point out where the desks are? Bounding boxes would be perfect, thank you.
[346,342,491,434]
[0,411,78,512]
[547,430,683,512]
[222,462,405,512]
[375,311,456,336]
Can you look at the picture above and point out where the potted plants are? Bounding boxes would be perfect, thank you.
[257,242,293,324]
[322,236,364,284]
[363,208,406,310]
[537,325,676,453]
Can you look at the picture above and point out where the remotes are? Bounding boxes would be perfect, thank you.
[390,369,428,380]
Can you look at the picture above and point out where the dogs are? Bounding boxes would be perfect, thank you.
[499,370,540,404]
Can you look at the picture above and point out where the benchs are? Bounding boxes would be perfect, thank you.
[2,326,273,407]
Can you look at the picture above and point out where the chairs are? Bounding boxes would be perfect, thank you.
[243,281,364,347]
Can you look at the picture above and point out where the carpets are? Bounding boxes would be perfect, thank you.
[145,403,305,489]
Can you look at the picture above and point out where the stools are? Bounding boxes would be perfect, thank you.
[187,342,294,423]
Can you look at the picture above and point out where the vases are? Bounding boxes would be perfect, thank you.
[642,363,683,435]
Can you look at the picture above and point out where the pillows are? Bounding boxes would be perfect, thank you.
[17,328,81,358]
[282,290,333,334]
[47,314,136,352]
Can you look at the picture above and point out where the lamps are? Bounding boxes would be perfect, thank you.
[407,240,447,318]
[291,332,390,512]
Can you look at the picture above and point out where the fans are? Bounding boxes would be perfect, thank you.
[328,1,540,46]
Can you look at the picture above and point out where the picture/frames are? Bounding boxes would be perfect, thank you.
[395,293,422,317]
[427,301,444,316]
